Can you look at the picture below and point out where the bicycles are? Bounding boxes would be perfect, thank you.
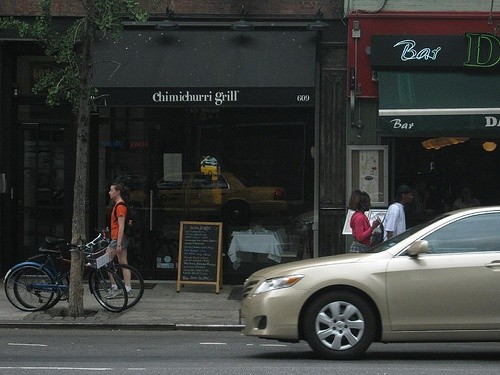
[3,228,145,313]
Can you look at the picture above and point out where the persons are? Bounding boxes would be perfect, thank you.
[104,181,134,299]
[382,185,414,242]
[451,184,481,210]
[350,191,381,254]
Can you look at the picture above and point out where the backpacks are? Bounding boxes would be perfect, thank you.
[114,199,143,237]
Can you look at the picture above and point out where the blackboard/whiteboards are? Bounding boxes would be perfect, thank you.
[178,221,222,284]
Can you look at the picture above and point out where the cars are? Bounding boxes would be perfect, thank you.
[105,170,290,231]
[241,204,500,361]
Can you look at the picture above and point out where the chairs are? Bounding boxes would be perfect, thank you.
[280,229,306,263]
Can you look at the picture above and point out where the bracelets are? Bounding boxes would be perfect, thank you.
[117,244,121,246]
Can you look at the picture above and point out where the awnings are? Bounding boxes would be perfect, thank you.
[376,69,500,140]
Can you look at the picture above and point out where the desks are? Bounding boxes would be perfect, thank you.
[227,231,284,272]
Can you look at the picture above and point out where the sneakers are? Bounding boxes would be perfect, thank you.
[116,289,134,298]
[105,287,122,298]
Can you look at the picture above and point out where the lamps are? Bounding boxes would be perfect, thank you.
[306,8,329,31]
[421,137,470,150]
[230,5,254,31]
[483,141,496,152]
[154,6,178,30]
